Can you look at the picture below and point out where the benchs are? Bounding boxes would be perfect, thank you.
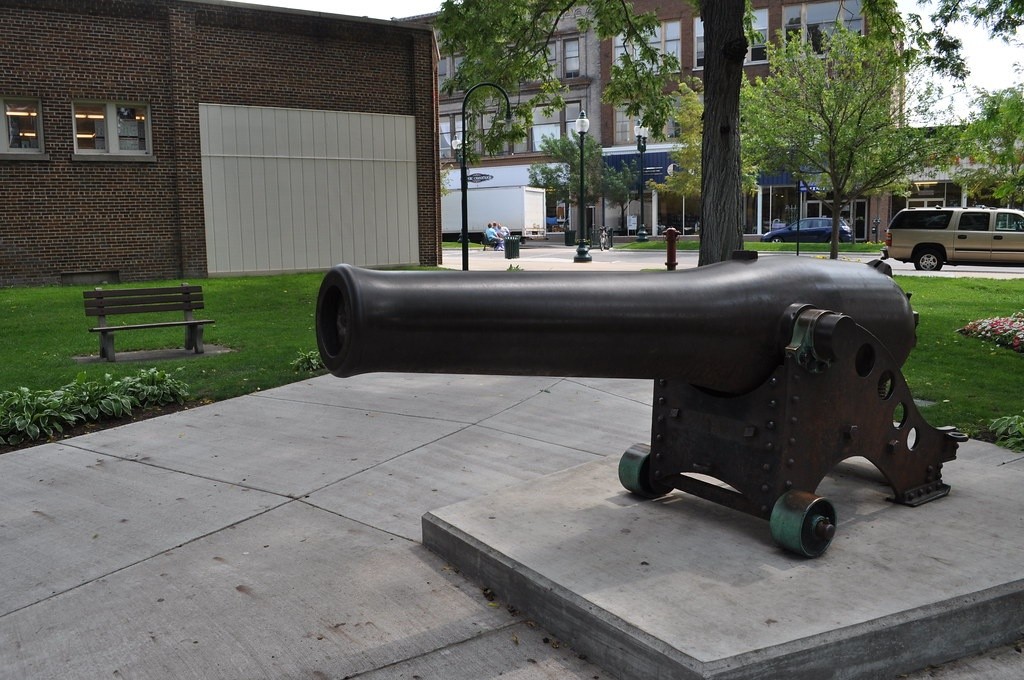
[482,233,499,250]
[83,282,215,364]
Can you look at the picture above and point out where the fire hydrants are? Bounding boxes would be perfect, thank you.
[664,227,680,269]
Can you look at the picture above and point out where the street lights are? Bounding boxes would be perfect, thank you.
[461,82,512,271]
[633,119,649,241]
[574,110,592,263]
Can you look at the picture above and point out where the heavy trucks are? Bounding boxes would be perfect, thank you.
[441,185,547,243]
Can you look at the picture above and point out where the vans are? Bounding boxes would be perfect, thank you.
[880,205,1024,271]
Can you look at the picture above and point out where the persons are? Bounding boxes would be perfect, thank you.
[496,223,510,249]
[486,223,505,251]
[492,222,498,234]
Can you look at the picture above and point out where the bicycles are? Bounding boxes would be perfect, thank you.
[598,225,610,251]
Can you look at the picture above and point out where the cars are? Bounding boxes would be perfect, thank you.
[760,217,852,243]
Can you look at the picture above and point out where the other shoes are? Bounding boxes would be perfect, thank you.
[498,247,505,251]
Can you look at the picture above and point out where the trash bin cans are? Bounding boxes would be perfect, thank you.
[565,230,577,246]
[502,235,521,259]
